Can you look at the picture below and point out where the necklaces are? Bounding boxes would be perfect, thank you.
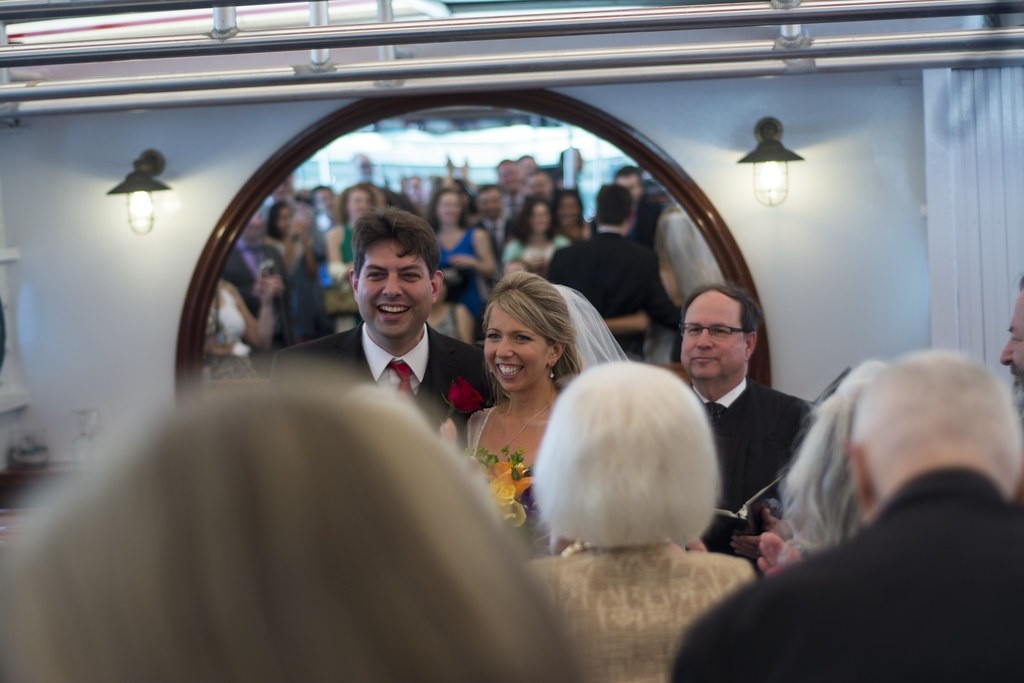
[501,401,553,454]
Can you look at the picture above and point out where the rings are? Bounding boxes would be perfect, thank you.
[755,551,760,555]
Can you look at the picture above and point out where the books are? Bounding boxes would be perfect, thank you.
[703,473,786,573]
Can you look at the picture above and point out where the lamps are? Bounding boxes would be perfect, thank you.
[734,117,805,208]
[105,148,171,236]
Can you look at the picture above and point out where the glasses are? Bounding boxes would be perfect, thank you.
[678,321,749,338]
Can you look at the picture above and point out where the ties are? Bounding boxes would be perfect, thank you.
[387,361,413,394]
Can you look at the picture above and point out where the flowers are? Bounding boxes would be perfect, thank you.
[463,446,550,560]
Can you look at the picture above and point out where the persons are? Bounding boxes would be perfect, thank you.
[679,282,817,579]
[200,154,728,382]
[0,278,1024,683]
[270,206,495,457]
[465,270,580,560]
